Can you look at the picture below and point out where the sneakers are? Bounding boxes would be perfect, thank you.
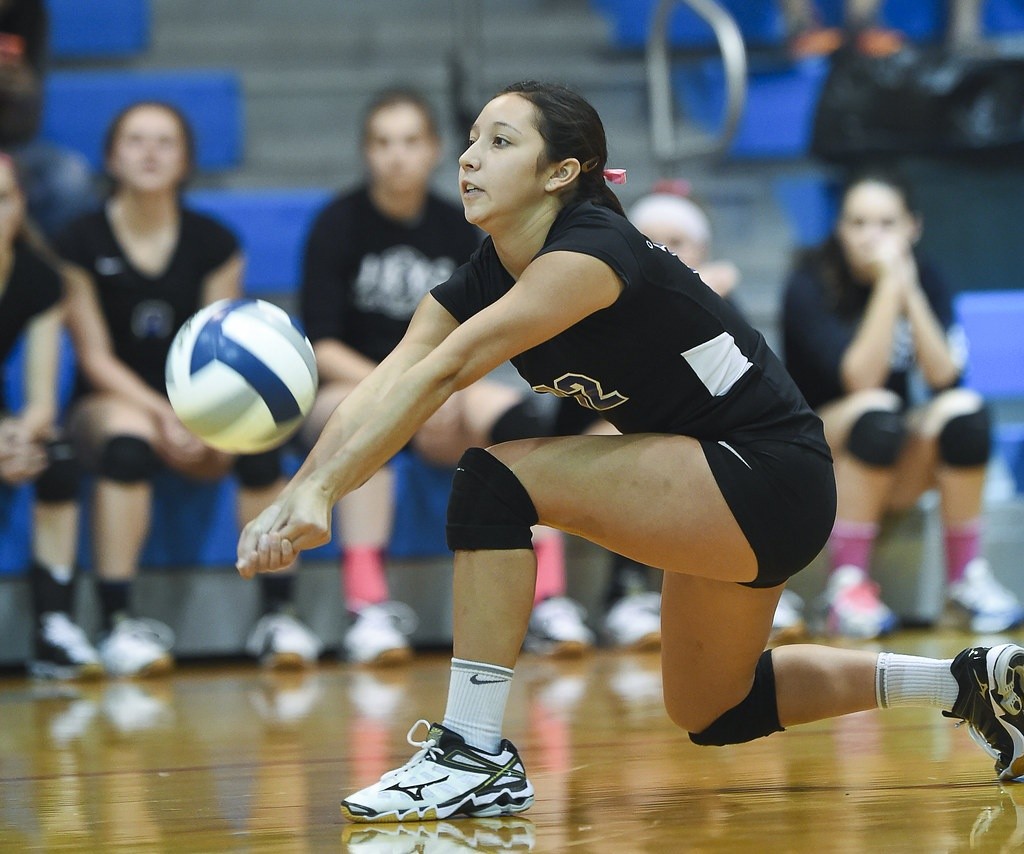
[943,555,1023,634]
[942,642,1024,781]
[341,718,536,823]
[340,813,536,854]
[960,783,1024,854]
[826,565,899,642]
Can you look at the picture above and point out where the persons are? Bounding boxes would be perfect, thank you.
[237,79,1023,818]
[776,171,1023,641]
[557,192,804,653]
[0,0,105,681]
[48,100,322,679]
[292,81,597,670]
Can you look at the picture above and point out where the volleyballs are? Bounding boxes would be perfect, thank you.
[165,297,319,456]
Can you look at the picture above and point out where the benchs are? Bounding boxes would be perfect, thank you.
[0,0,476,676]
[592,0,1024,621]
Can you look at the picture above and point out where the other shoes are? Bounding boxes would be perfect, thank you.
[247,611,320,671]
[768,589,812,643]
[95,616,175,679]
[524,595,596,659]
[28,616,105,682]
[337,602,415,666]
[600,594,663,650]
